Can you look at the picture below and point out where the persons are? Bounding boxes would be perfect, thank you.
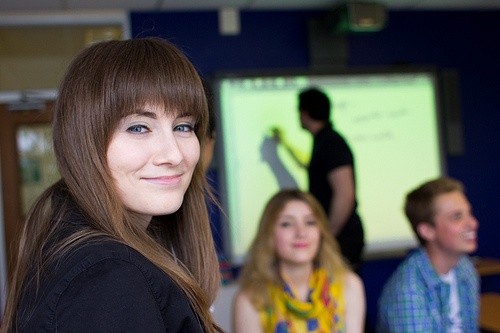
[375,178,480,333]
[0,35,221,333]
[231,188,366,332]
[272,85,365,278]
